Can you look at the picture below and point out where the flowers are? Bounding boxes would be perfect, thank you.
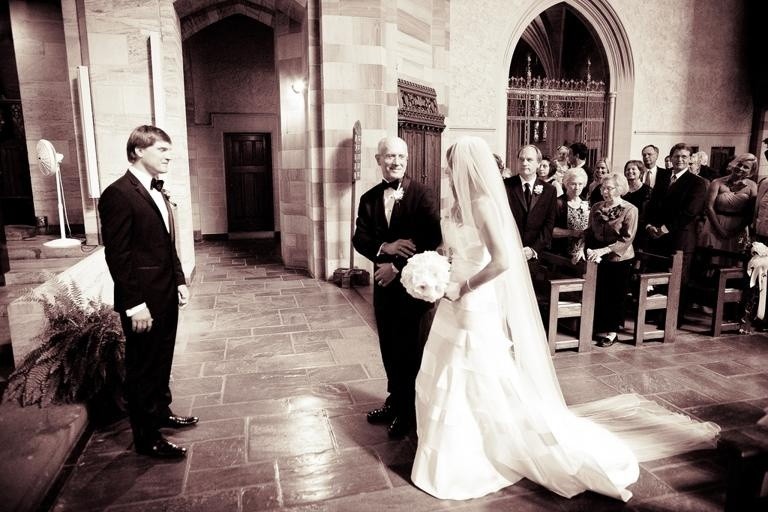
[394,248,453,305]
[532,184,545,195]
[580,200,589,214]
[162,188,176,201]
[389,186,405,202]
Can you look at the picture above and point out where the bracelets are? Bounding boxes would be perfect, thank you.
[465,278,476,291]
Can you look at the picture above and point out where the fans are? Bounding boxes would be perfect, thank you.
[31,135,84,254]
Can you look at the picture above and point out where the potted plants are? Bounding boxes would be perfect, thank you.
[0,268,126,434]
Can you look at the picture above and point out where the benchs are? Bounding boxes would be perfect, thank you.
[707,248,759,340]
[630,241,689,346]
[536,226,602,357]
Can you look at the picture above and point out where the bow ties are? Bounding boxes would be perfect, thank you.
[382,179,400,191]
[150,178,164,190]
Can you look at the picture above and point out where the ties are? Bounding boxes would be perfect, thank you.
[669,176,676,187]
[645,171,650,188]
[524,183,532,207]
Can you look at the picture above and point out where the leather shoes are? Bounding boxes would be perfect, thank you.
[136,436,187,459]
[367,404,415,436]
[601,334,618,347]
[162,413,199,428]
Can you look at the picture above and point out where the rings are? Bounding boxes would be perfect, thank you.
[378,281,382,286]
[400,246,402,251]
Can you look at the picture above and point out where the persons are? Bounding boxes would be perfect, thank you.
[353,135,443,443]
[407,135,544,499]
[621,142,719,330]
[739,137,768,335]
[692,153,759,316]
[493,142,638,349]
[97,124,201,460]
